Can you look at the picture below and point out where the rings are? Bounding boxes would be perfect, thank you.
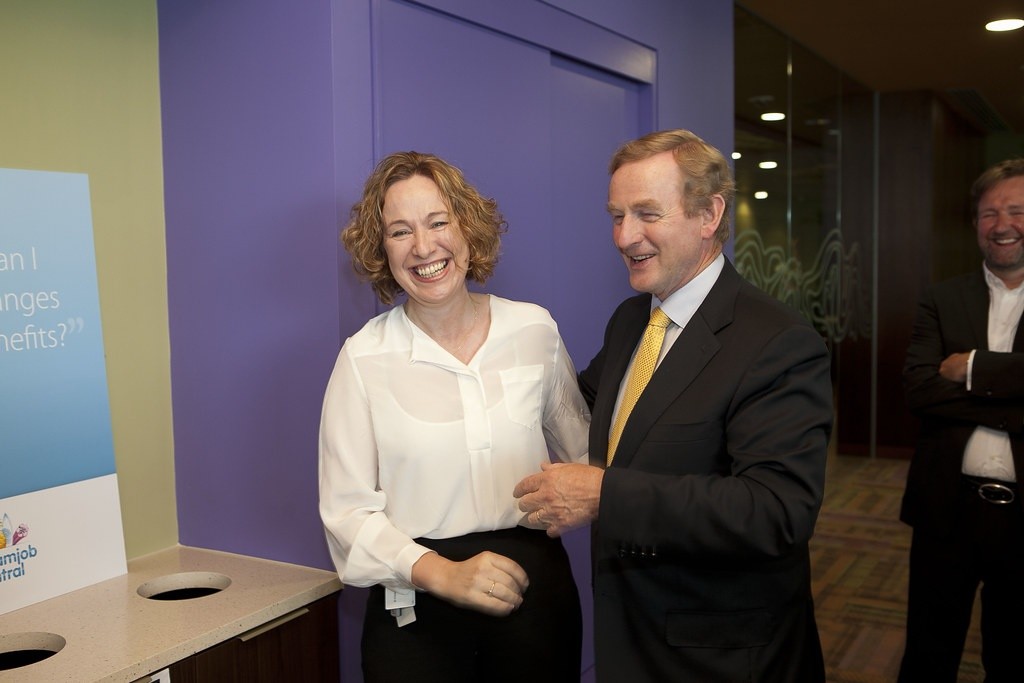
[536,512,543,523]
[489,584,495,595]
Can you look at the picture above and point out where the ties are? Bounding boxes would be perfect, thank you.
[604,306,672,465]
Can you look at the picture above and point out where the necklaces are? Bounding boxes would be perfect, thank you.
[412,296,477,355]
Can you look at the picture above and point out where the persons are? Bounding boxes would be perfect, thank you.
[510,130,835,682]
[895,157,1023,683]
[318,150,592,682]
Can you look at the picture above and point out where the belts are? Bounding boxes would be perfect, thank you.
[961,477,1018,506]
